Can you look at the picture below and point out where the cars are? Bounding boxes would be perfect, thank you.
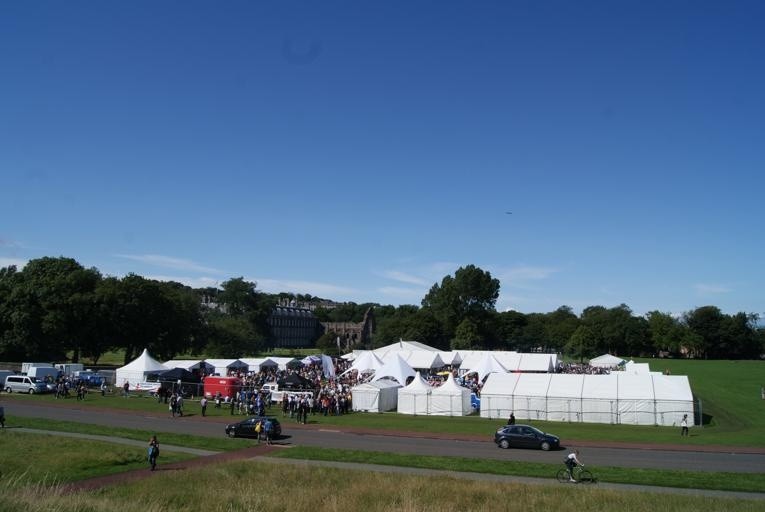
[36,379,57,393]
[81,373,106,385]
[494,424,560,451]
[225,417,281,439]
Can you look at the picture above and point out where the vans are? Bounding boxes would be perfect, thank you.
[4,375,47,395]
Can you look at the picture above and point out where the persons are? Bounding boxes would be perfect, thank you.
[563,448,585,483]
[507,412,516,425]
[679,414,689,437]
[254,418,264,443]
[427,366,480,397]
[124,381,130,396]
[148,436,160,471]
[263,418,274,446]
[100,381,106,396]
[158,358,371,425]
[0,407,5,429]
[554,361,626,377]
[43,372,88,401]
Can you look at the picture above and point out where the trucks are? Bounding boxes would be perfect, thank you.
[27,367,63,378]
[260,382,316,405]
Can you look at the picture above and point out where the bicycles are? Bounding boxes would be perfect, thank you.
[556,464,593,485]
